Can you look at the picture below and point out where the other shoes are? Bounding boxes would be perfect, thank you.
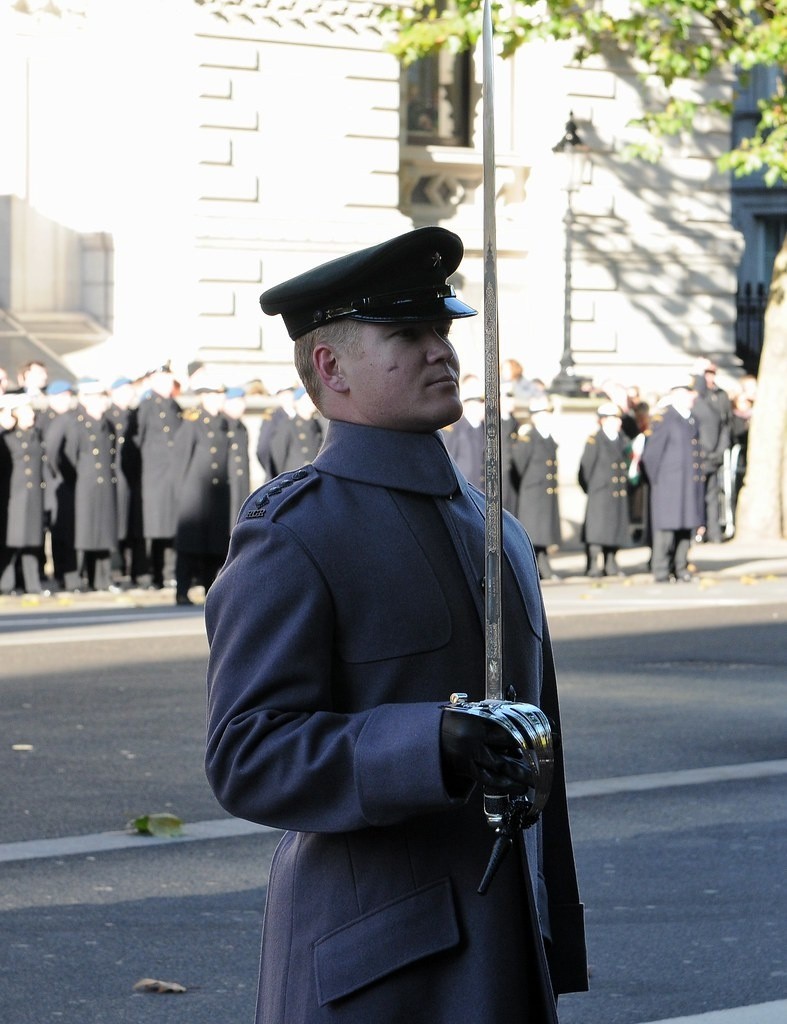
[655,575,700,583]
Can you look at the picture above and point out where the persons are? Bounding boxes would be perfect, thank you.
[1,358,749,610]
[203,228,591,1024]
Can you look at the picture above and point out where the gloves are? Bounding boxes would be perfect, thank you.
[440,704,563,796]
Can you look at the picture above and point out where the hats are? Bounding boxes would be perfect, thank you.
[111,378,132,390]
[225,387,245,398]
[46,380,74,394]
[140,389,153,402]
[295,387,306,400]
[259,227,479,342]
[596,404,624,416]
[78,376,100,383]
